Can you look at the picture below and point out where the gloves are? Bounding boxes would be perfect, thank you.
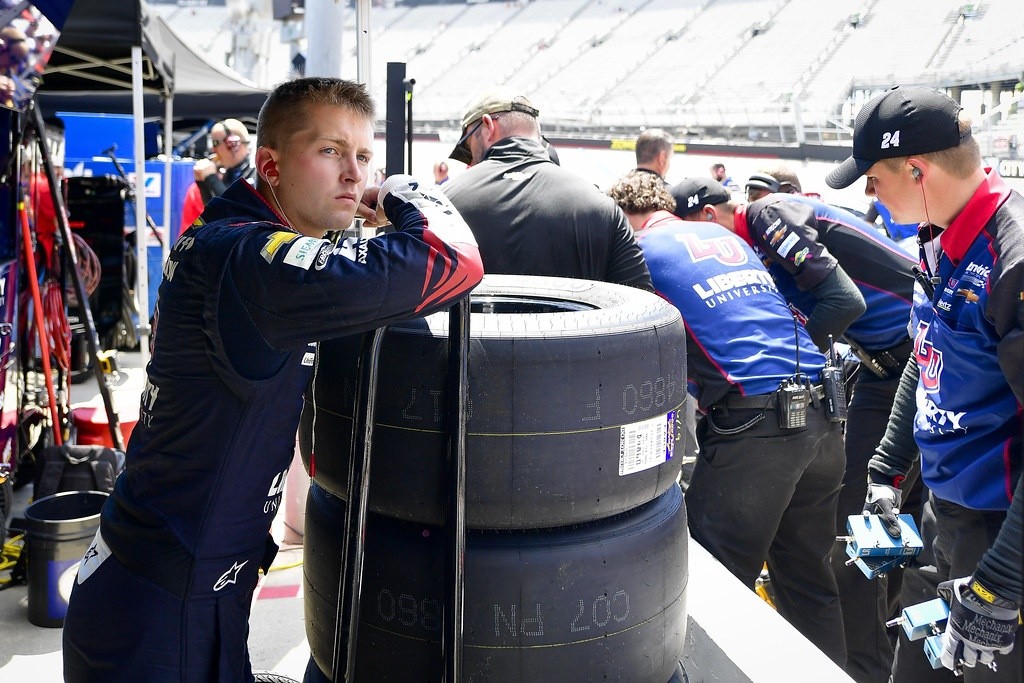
[936,576,1022,670]
[861,459,907,538]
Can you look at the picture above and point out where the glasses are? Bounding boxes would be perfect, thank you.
[213,140,224,146]
[458,114,499,155]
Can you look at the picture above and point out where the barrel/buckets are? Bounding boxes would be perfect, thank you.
[23,490,111,628]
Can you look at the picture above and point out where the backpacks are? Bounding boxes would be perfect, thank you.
[34,447,128,505]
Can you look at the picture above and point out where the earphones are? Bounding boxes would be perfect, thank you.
[263,160,279,176]
[707,214,713,220]
[913,168,921,178]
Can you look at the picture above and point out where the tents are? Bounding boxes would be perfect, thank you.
[140,13,272,128]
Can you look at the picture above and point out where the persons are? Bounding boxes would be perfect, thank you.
[191,118,256,208]
[434,92,1024,683]
[63,77,484,683]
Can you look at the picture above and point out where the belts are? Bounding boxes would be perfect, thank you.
[867,339,913,369]
[713,383,825,410]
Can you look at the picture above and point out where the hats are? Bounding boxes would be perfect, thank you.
[448,95,539,166]
[825,85,972,189]
[670,176,731,217]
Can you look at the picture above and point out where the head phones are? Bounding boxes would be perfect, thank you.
[220,120,241,151]
[744,173,798,194]
